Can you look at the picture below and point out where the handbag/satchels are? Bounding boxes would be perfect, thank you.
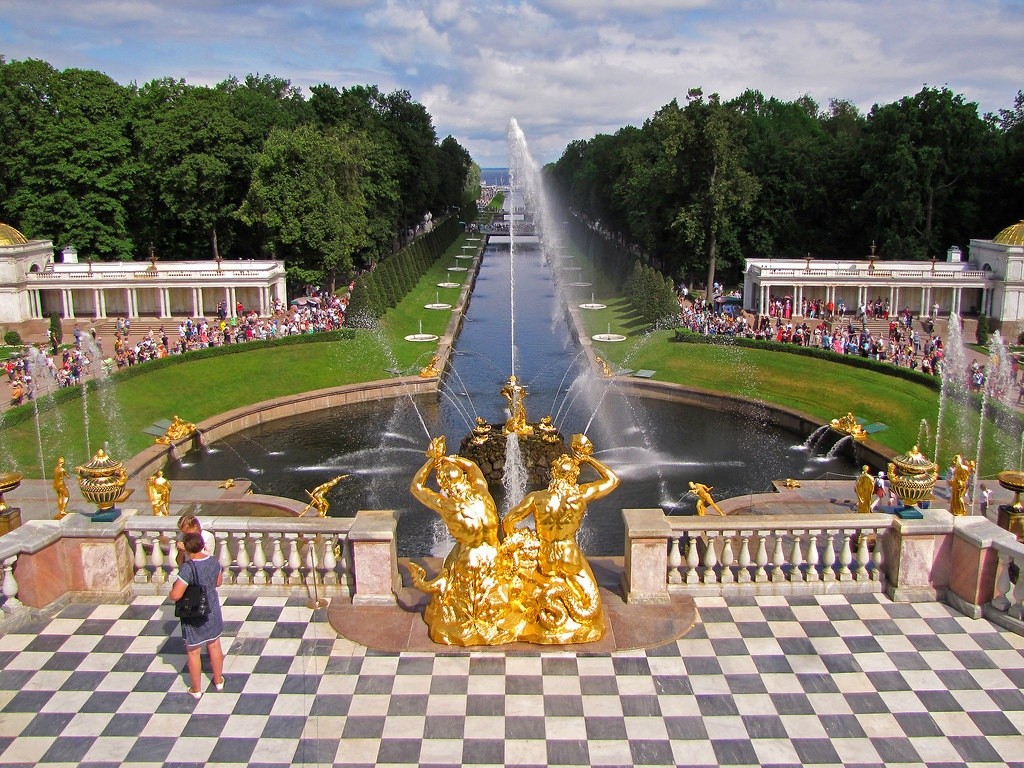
[175,558,212,617]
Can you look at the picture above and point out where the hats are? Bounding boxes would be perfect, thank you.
[878,471,884,478]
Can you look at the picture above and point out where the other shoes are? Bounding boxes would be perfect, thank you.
[214,675,225,690]
[870,506,873,513]
[187,686,201,700]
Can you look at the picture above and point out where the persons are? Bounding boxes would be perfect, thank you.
[168,515,227,699]
[980,483,994,518]
[5,279,358,407]
[869,471,887,513]
[675,282,1024,404]
